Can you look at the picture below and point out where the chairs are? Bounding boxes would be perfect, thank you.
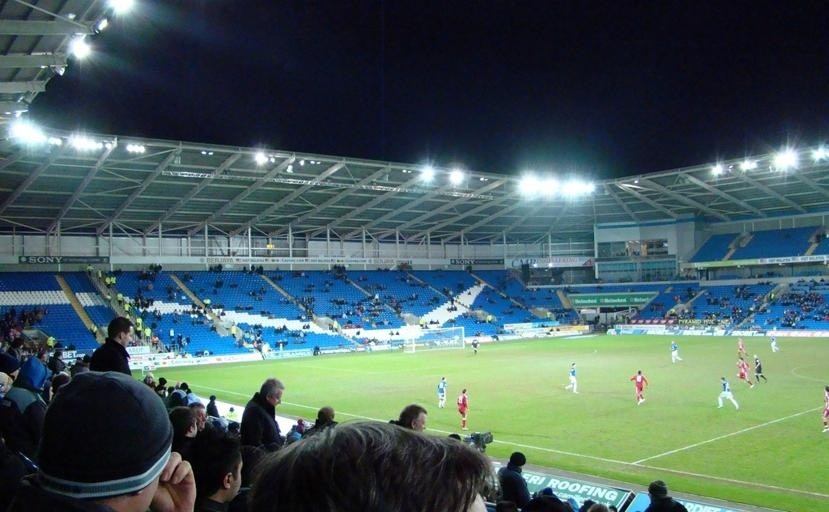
[618,224,828,330]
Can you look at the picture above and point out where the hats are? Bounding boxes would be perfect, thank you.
[34,370,189,499]
[648,481,667,496]
[0,352,21,374]
[510,452,526,466]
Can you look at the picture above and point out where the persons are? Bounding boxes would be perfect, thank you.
[1,248,829,512]
[816,232,822,242]
